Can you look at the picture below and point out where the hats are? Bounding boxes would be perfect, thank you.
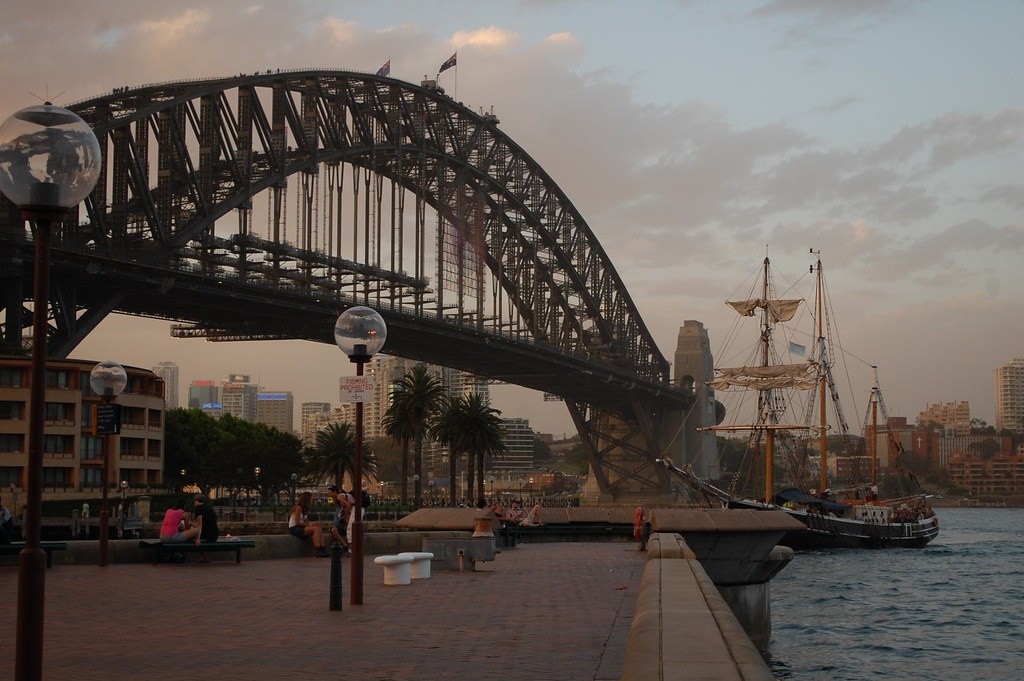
[192,494,206,502]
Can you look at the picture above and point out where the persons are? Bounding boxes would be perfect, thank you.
[633,506,646,543]
[328,485,365,557]
[193,494,218,563]
[806,505,836,517]
[891,500,932,522]
[487,498,544,527]
[289,491,333,558]
[0,496,13,543]
[830,494,836,503]
[160,499,199,542]
[871,482,878,501]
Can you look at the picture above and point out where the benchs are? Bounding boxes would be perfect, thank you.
[499,522,650,551]
[0,543,67,569]
[138,539,255,564]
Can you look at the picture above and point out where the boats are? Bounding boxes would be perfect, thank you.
[652,246,943,548]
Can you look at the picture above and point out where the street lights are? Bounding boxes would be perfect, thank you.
[529,478,535,508]
[488,476,495,507]
[120,480,129,521]
[0,102,103,681]
[380,481,385,502]
[179,469,187,504]
[87,361,129,569]
[517,479,523,507]
[332,305,388,606]
[253,466,261,506]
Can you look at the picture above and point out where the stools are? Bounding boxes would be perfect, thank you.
[373,551,434,585]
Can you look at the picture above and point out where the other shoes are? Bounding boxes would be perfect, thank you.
[198,559,209,563]
[316,546,331,558]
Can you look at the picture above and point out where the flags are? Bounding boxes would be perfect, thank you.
[376,60,390,77]
[439,53,456,73]
